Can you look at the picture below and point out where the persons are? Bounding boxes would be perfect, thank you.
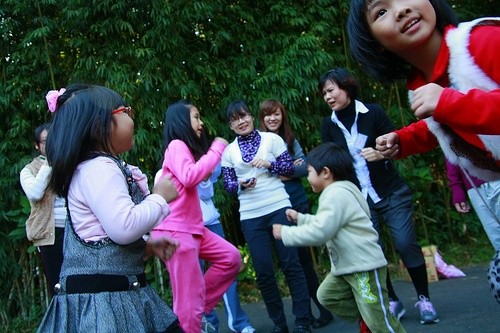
[346,0,500,252]
[19,82,257,333]
[317,68,441,324]
[272,141,406,333]
[257,99,335,329]
[220,100,316,333]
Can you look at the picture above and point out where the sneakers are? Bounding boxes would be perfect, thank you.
[388,299,407,320]
[241,326,256,333]
[414,294,440,323]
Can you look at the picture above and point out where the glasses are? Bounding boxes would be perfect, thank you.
[229,111,252,124]
[111,106,133,118]
[40,140,46,144]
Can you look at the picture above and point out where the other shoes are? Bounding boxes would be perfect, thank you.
[312,310,334,327]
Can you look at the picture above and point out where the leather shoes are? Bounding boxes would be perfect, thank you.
[274,325,289,333]
[294,323,312,333]
